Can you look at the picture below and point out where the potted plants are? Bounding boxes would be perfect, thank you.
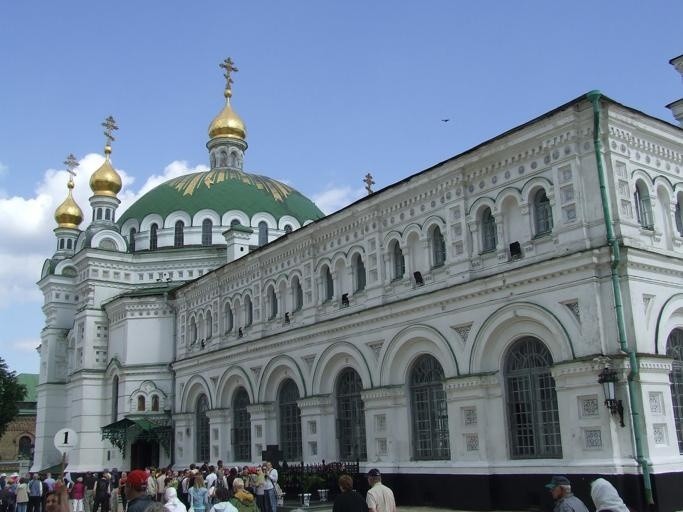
[315,466,340,506]
[295,468,317,509]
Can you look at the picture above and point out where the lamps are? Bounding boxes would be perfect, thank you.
[596,362,627,429]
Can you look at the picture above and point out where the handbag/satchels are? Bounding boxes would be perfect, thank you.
[187,487,195,512]
[273,483,283,499]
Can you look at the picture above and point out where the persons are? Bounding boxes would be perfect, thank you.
[332,474,368,512]
[366,469,397,512]
[590,478,630,512]
[0,460,284,512]
[544,475,590,512]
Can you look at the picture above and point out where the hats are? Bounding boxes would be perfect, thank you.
[120,470,148,488]
[544,476,570,488]
[364,469,380,477]
